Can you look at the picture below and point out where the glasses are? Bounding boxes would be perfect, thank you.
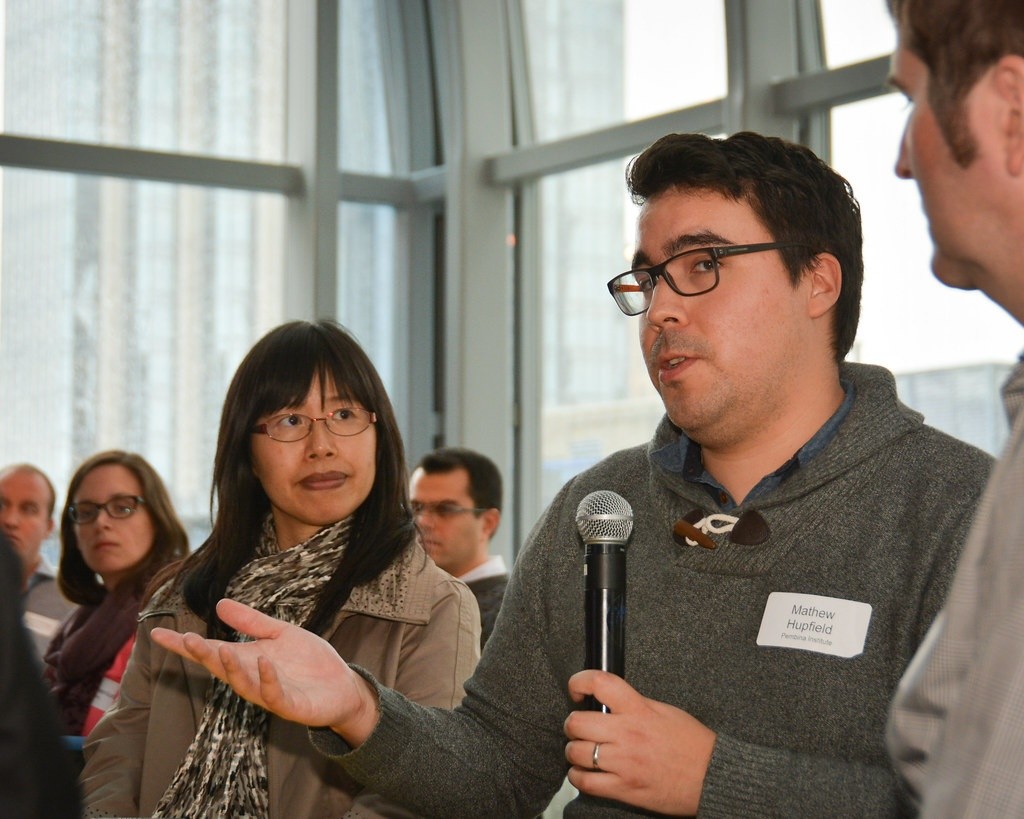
[607,242,825,316]
[250,408,377,443]
[68,495,146,524]
[411,503,489,518]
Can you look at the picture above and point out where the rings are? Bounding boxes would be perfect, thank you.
[593,743,600,771]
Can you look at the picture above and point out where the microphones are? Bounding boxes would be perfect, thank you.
[574,489,635,713]
